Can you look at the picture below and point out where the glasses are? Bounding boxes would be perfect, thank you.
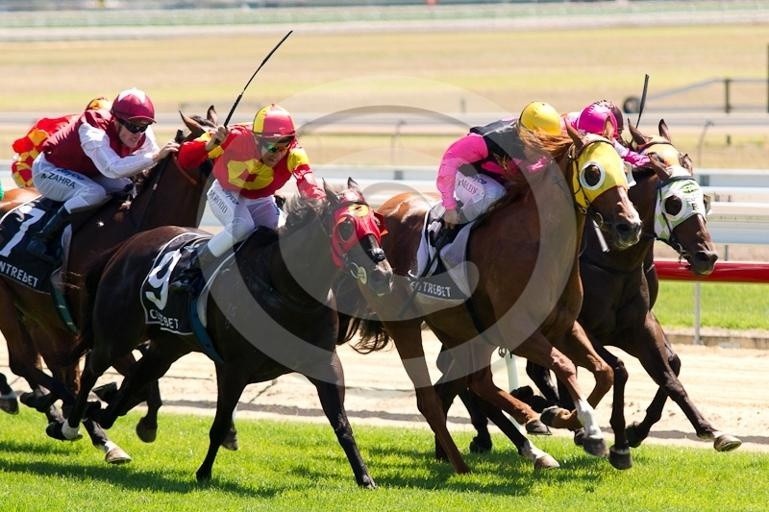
[117,117,148,134]
[258,140,291,153]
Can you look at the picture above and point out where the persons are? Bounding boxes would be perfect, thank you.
[176,100,329,299]
[568,103,653,176]
[593,100,635,151]
[9,95,114,188]
[31,87,179,268]
[435,100,564,233]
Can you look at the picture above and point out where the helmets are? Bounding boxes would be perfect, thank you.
[514,100,563,153]
[251,104,296,140]
[82,88,158,124]
[575,99,625,136]
[166,242,219,315]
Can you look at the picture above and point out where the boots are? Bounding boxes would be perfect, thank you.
[433,209,470,250]
[25,205,72,267]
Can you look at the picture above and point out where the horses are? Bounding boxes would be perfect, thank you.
[0,102,285,465]
[430,151,742,463]
[45,177,394,491]
[329,117,645,473]
[509,119,711,449]
